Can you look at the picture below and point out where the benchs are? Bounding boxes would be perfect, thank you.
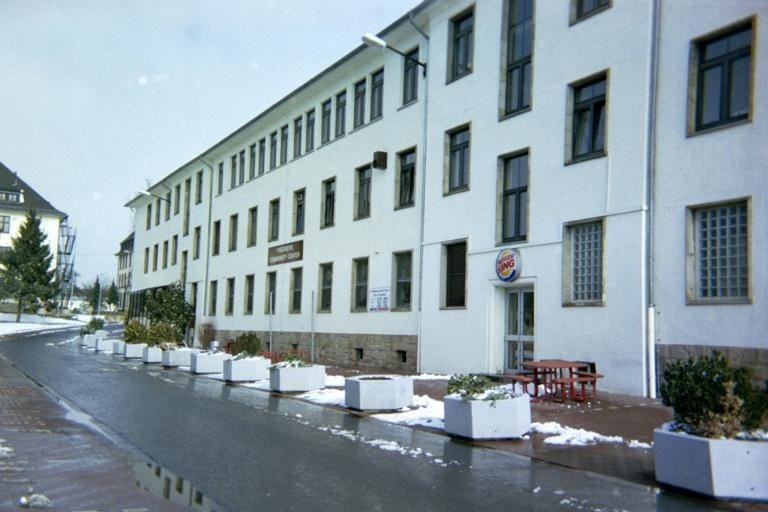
[503,358,603,403]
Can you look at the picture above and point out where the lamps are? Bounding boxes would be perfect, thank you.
[133,186,170,205]
[359,31,426,78]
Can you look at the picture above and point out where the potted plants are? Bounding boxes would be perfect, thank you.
[269,351,325,394]
[443,373,530,440]
[142,321,182,364]
[648,347,766,503]
[222,331,264,383]
[124,319,146,358]
[78,317,105,349]
